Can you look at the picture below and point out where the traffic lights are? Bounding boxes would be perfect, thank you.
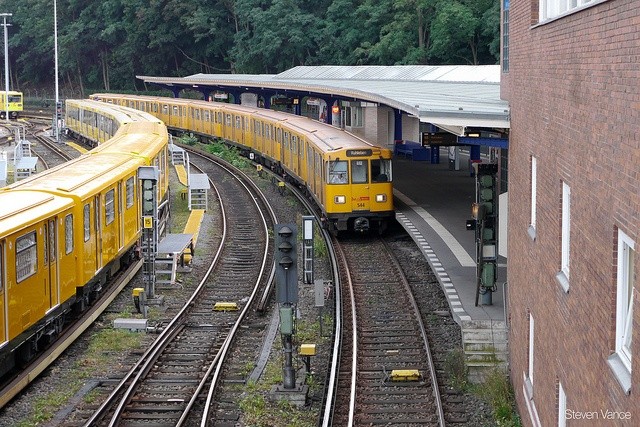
[57,102,62,120]
[273,223,298,268]
[472,202,487,220]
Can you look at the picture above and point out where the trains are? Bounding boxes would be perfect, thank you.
[0,91,23,120]
[0,99,170,390]
[89,92,395,239]
